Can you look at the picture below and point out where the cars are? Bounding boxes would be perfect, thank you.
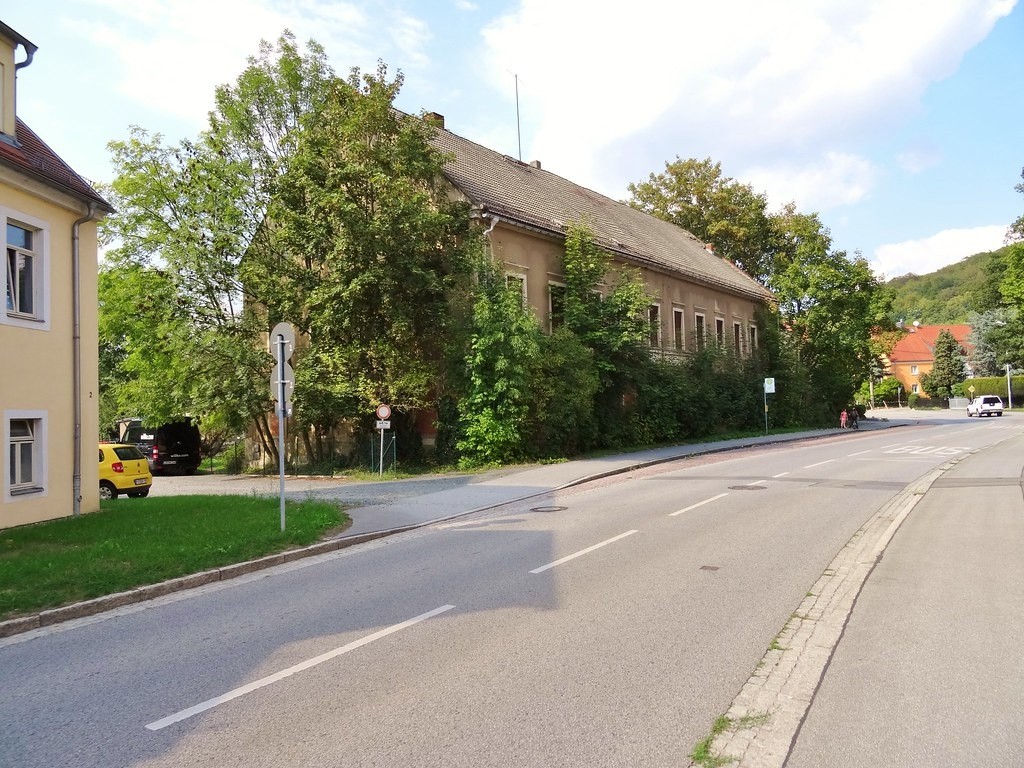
[967,395,1004,417]
[98,444,153,500]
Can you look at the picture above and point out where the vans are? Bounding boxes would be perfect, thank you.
[119,416,201,476]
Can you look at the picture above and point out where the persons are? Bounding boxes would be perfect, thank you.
[848,407,859,429]
[840,408,848,429]
